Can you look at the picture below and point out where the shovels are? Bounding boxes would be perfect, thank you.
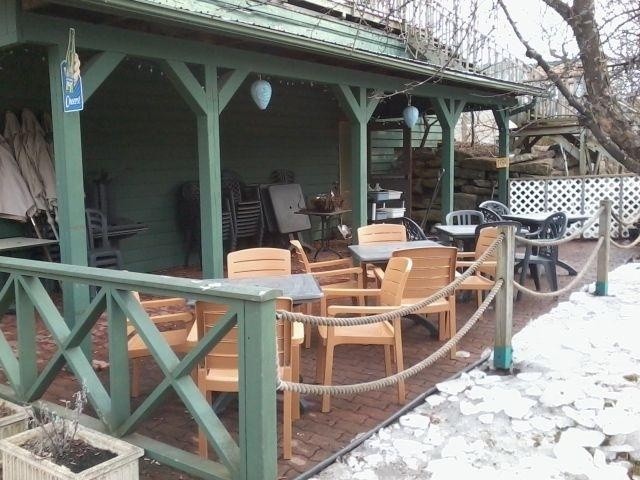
[337,214,352,240]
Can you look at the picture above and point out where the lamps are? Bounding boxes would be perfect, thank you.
[402,93,420,130]
[248,74,274,110]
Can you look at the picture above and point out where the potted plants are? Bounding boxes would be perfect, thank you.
[0,376,149,479]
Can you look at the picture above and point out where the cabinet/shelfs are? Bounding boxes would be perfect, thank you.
[369,186,407,223]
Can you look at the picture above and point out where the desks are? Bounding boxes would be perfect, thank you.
[346,240,446,337]
[185,272,325,417]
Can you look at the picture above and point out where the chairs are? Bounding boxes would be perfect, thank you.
[290,239,367,349]
[435,200,578,304]
[373,246,459,367]
[182,174,353,268]
[443,227,499,340]
[401,216,445,246]
[314,256,413,413]
[356,223,407,283]
[227,247,291,279]
[195,297,294,464]
[0,208,151,314]
[119,269,194,398]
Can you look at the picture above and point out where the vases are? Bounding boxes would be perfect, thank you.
[0,397,33,471]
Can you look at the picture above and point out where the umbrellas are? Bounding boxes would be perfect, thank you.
[1,54,61,293]
